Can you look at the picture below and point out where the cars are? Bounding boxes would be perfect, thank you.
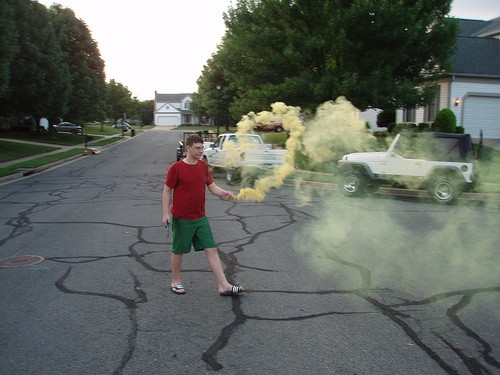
[251,116,303,132]
[176,131,213,163]
[112,122,131,129]
[53,122,81,134]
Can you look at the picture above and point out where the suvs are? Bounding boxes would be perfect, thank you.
[336,131,475,206]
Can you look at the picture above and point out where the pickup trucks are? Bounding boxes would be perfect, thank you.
[200,134,288,186]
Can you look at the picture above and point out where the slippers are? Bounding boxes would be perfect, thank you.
[220,286,244,296]
[170,283,186,294]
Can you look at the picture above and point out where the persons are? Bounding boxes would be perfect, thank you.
[162,135,245,297]
